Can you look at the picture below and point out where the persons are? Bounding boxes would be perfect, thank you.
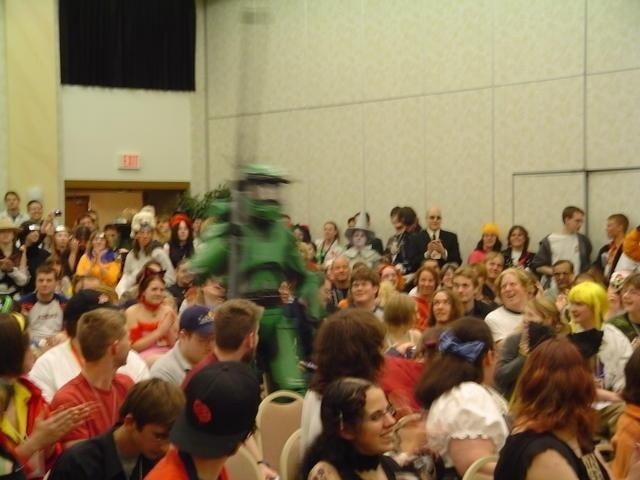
[1,156,640,480]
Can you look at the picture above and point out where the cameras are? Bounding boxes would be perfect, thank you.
[54,208,63,217]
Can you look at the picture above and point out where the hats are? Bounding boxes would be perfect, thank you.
[0,219,21,231]
[180,305,215,337]
[482,224,498,234]
[170,212,192,227]
[169,361,258,458]
[63,290,119,319]
[344,208,375,241]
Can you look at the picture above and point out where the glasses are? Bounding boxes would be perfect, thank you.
[430,216,441,220]
[369,406,394,421]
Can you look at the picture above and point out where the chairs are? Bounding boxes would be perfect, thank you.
[462,455,498,479]
[255,389,305,473]
[223,446,260,480]
[280,429,302,480]
